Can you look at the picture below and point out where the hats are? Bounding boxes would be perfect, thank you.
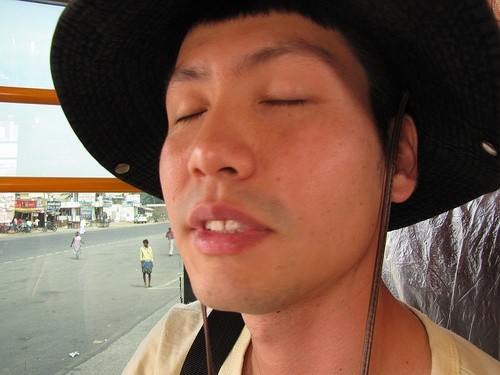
[51,0,500,232]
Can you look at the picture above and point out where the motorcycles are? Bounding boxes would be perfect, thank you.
[42,221,57,233]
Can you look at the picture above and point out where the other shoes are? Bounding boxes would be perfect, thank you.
[169,253,174,256]
[148,286,152,287]
[145,285,147,288]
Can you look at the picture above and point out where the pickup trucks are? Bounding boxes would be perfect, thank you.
[133,214,147,223]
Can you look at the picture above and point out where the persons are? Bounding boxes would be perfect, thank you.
[138,238,156,288]
[69,232,84,261]
[49,0,499,374]
[165,227,175,256]
